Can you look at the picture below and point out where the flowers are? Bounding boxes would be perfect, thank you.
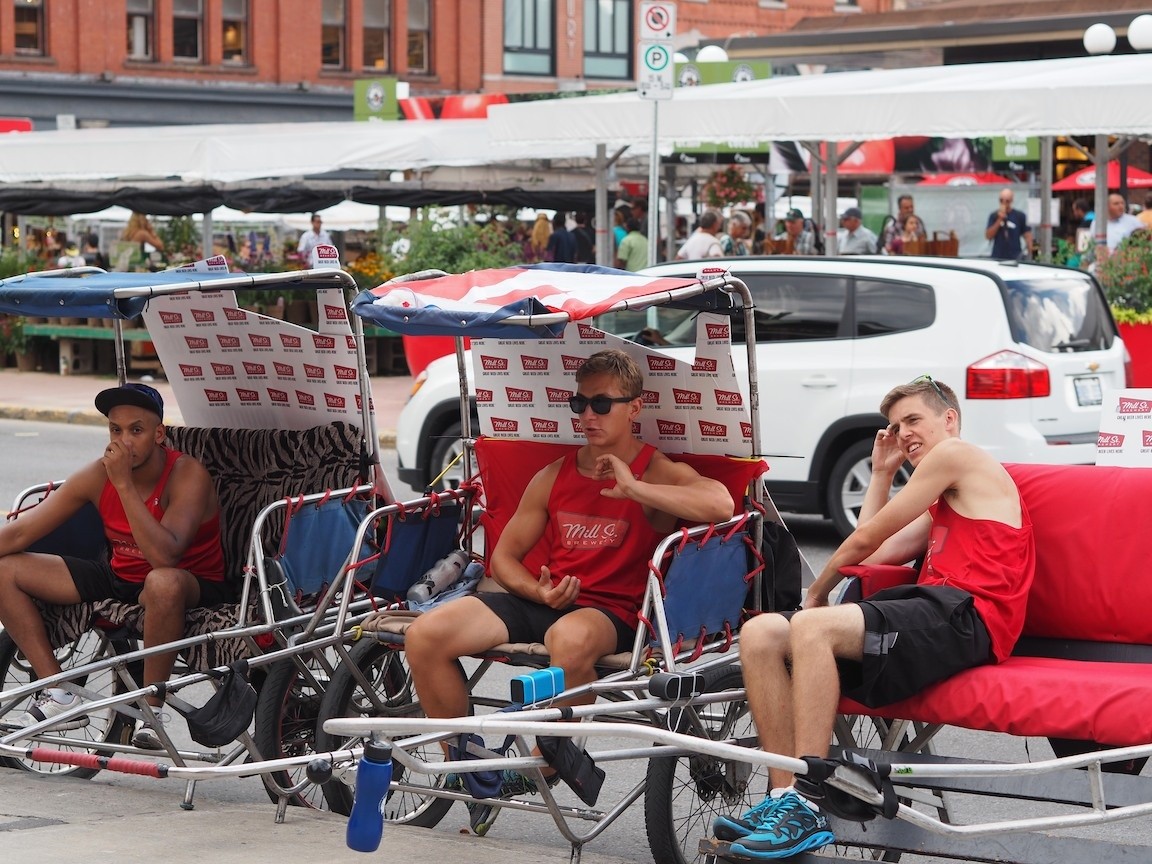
[699,161,766,209]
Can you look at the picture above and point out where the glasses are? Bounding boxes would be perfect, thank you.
[1000,198,1011,203]
[907,374,953,409]
[568,395,634,415]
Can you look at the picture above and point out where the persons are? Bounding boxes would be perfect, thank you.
[399,346,738,842]
[1,378,224,754]
[1,189,1152,346]
[708,376,1040,862]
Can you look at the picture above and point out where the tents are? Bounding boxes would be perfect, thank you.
[0,94,632,266]
[484,52,1151,277]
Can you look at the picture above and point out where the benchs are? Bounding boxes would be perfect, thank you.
[358,430,766,673]
[17,422,368,677]
[830,458,1152,750]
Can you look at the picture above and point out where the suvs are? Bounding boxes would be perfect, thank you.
[392,252,1125,541]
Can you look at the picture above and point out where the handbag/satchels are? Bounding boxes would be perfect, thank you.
[186,670,259,749]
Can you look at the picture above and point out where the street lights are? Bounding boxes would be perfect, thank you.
[1083,21,1114,265]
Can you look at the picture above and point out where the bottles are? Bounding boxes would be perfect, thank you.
[345,732,392,852]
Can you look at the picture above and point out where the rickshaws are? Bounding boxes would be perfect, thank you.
[308,261,815,863]
[333,388,1150,863]
[0,254,403,800]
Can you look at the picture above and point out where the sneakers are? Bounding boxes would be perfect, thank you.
[445,773,511,837]
[131,710,167,750]
[459,768,561,799]
[711,793,779,843]
[729,791,836,861]
[0,691,90,732]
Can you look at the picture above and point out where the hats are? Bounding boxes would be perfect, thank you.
[839,208,863,220]
[783,208,804,221]
[94,382,164,424]
